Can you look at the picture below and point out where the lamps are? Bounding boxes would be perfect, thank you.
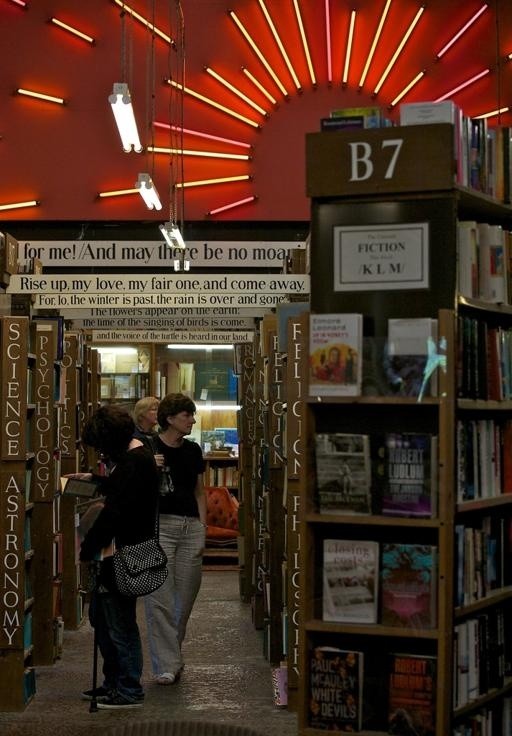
[161,47,189,248]
[173,254,189,274]
[109,0,146,152]
[134,28,163,212]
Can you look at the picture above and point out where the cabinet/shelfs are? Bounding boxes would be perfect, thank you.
[161,346,238,486]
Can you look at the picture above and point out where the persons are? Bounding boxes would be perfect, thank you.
[60,407,161,708]
[133,396,160,449]
[147,394,207,684]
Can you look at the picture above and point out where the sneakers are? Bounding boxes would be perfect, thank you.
[157,671,176,685]
[81,685,112,700]
[91,693,145,710]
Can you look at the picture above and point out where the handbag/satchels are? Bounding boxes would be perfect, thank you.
[113,536,170,599]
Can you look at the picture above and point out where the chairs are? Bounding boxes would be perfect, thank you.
[204,487,238,538]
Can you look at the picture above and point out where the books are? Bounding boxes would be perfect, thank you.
[453,704,512,736]
[456,316,512,401]
[307,646,363,734]
[311,432,439,519]
[451,611,510,713]
[455,514,511,608]
[320,107,397,130]
[456,220,510,305]
[400,102,511,203]
[384,653,438,734]
[321,540,439,629]
[386,318,437,398]
[22,309,105,706]
[457,418,511,502]
[308,313,364,397]
